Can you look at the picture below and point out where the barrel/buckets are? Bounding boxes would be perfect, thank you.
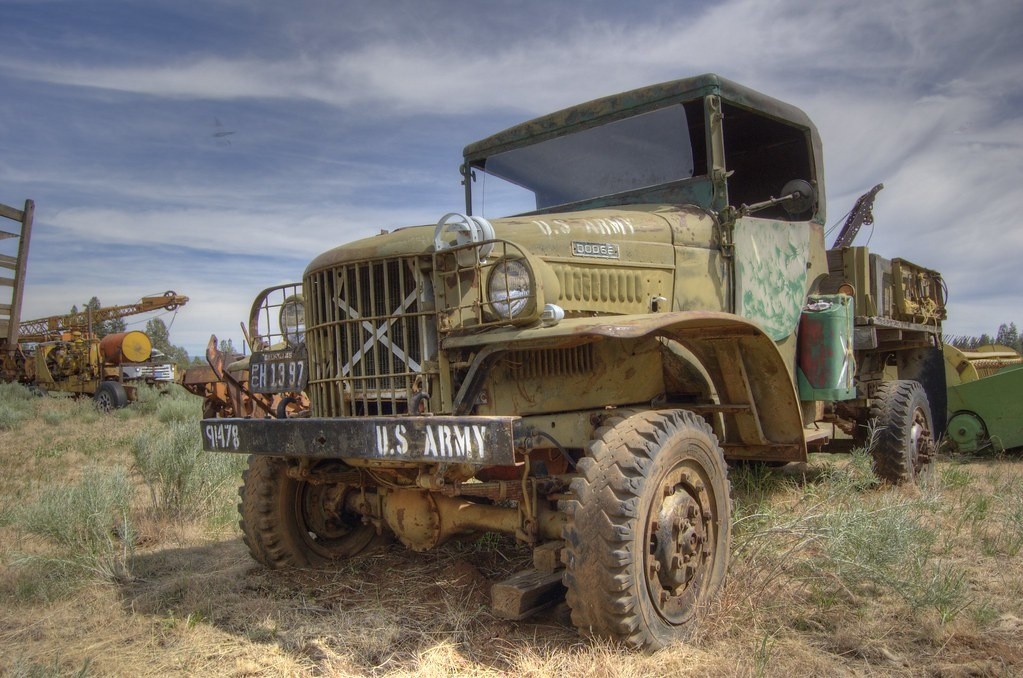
[797,293,857,402]
[99,331,152,364]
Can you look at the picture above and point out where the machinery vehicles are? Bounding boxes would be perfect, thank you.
[0,200,190,412]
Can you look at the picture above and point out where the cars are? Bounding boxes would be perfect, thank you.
[118,347,177,384]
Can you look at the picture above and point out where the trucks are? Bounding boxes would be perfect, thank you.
[181,71,949,659]
